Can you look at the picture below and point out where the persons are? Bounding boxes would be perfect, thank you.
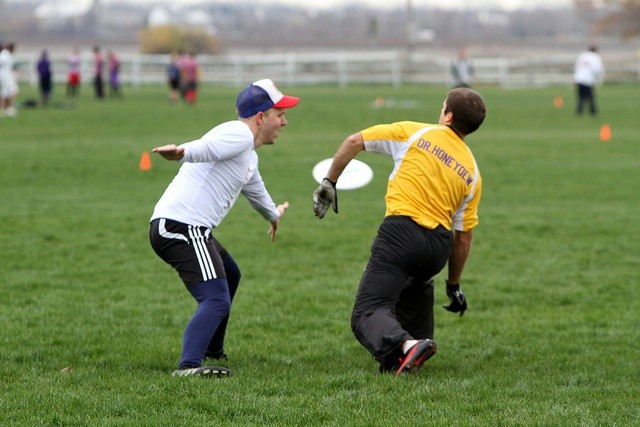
[573,43,605,115]
[0,42,20,116]
[179,51,200,102]
[38,48,54,105]
[448,48,476,89]
[313,85,487,375]
[151,78,300,379]
[108,50,121,95]
[92,41,106,97]
[68,43,80,96]
[167,52,179,100]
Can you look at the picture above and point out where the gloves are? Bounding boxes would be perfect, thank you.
[443,280,467,317]
[312,178,338,219]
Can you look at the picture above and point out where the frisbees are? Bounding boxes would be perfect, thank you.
[312,158,373,190]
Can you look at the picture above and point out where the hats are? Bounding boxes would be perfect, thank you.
[236,79,301,119]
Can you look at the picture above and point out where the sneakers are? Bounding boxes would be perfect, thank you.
[390,339,437,378]
[204,355,227,361]
[172,366,229,378]
[379,364,391,374]
